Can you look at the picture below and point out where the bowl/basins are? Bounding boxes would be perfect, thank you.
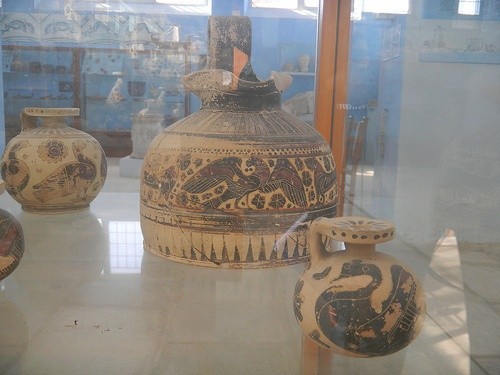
[127,81,145,97]
